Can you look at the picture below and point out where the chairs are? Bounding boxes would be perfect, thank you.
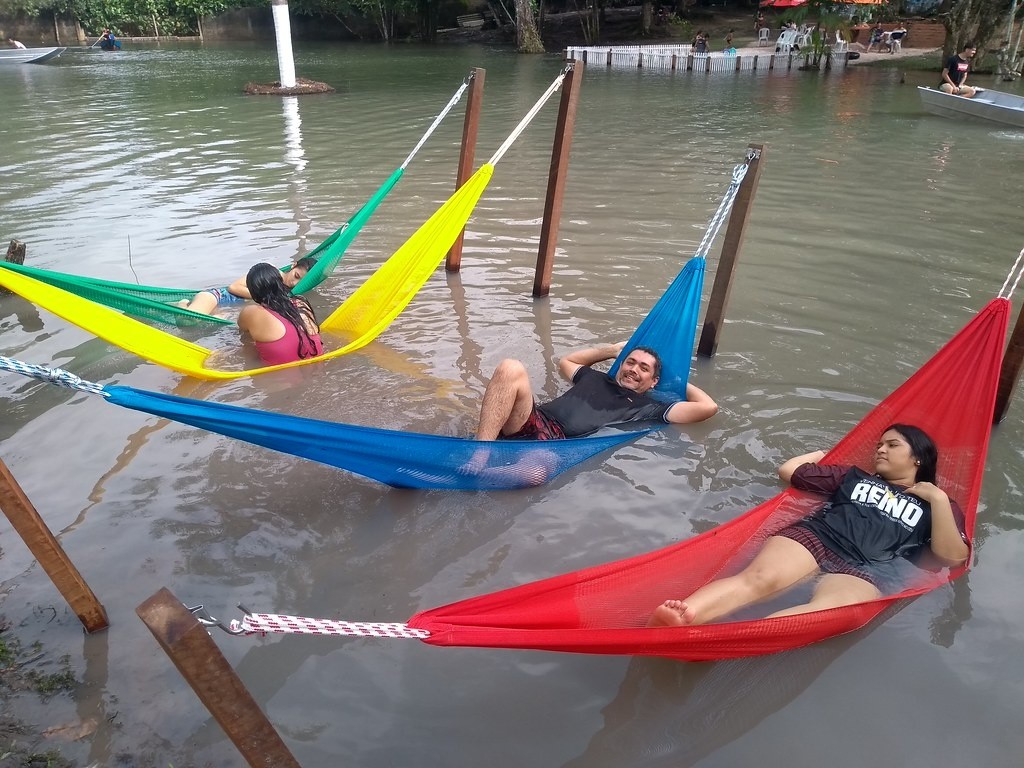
[758,24,850,52]
[871,32,903,53]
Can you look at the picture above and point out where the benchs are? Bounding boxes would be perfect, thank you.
[457,13,485,28]
[972,99,996,103]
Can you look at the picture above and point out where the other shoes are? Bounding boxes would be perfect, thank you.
[891,51,894,54]
[865,50,869,52]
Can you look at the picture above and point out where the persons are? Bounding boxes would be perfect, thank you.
[776,19,800,54]
[456,341,718,487]
[723,29,735,49]
[104,29,115,40]
[237,263,324,364]
[164,257,319,326]
[865,21,908,54]
[8,36,26,49]
[752,10,766,31]
[690,29,710,53]
[645,423,970,633]
[938,40,976,97]
